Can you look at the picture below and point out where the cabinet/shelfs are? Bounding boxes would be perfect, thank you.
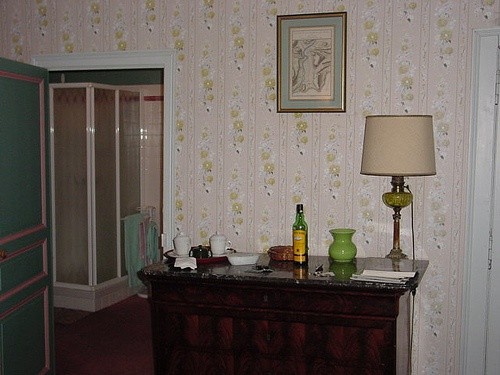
[136,251,431,375]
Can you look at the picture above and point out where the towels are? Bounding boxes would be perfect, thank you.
[125,206,158,287]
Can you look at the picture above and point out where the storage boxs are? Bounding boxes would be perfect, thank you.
[226,252,260,267]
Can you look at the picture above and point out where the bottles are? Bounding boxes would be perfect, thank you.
[329,228,357,262]
[292,204,308,266]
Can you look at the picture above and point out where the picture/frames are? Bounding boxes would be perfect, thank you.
[275,11,348,115]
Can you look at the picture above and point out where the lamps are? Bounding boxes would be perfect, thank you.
[359,113,436,261]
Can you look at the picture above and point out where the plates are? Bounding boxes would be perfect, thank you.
[227,254,259,265]
[167,251,193,257]
[208,250,234,257]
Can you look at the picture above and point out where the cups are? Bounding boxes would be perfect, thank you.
[189,245,213,258]
[209,231,232,255]
[173,231,191,255]
